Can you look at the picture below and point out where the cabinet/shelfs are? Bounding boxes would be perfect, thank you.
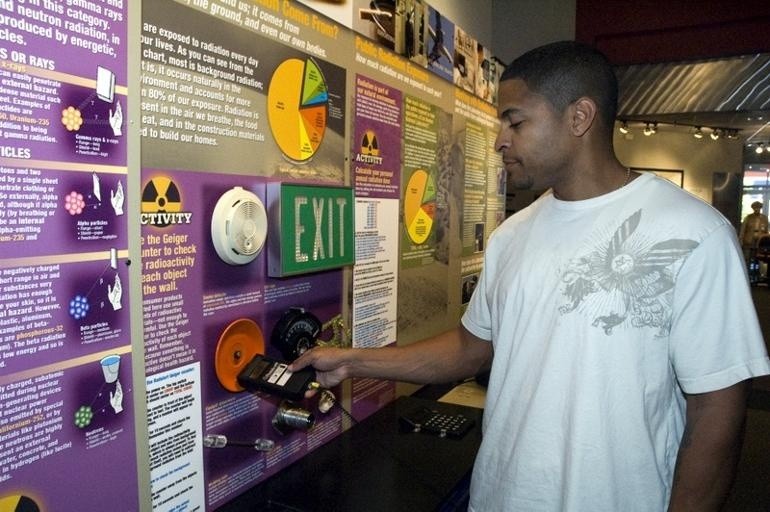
[217,396,487,512]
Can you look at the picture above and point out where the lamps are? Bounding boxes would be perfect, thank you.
[727,129,732,138]
[694,125,703,138]
[651,122,657,133]
[620,120,628,134]
[643,122,651,136]
[710,127,719,140]
[756,143,763,154]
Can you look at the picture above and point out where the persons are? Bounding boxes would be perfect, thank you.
[739,202,769,248]
[287,41,770,511]
